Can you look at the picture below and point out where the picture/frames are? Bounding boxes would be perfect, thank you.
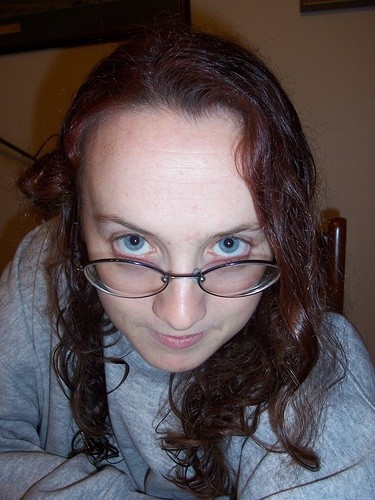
[0,0,188,56]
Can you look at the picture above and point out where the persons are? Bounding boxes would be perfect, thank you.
[0,33,375,499]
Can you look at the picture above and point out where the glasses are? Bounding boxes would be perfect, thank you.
[67,196,283,300]
